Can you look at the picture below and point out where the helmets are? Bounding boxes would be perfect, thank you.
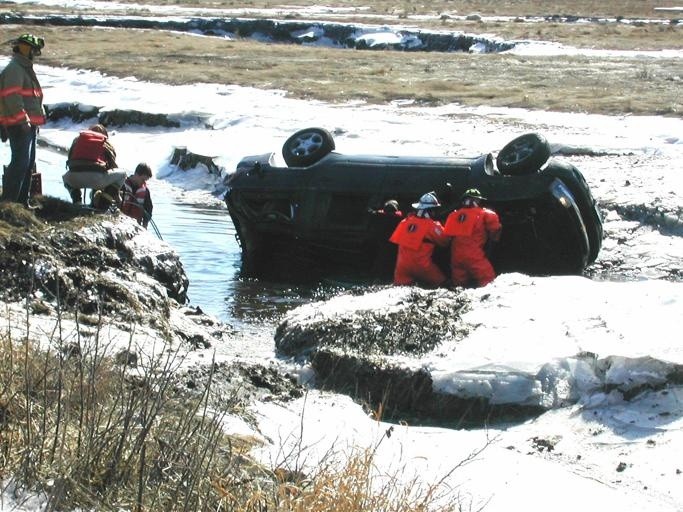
[412,193,441,210]
[462,187,487,202]
[18,33,44,51]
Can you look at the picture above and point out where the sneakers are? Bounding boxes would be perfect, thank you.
[72,196,82,206]
[99,200,112,209]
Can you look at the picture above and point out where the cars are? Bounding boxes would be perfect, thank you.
[222,126,603,288]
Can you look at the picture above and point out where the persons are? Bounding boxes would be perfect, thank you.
[114,162,153,229]
[62,123,127,212]
[388,190,447,285]
[366,199,403,276]
[0,33,48,210]
[445,188,502,287]
